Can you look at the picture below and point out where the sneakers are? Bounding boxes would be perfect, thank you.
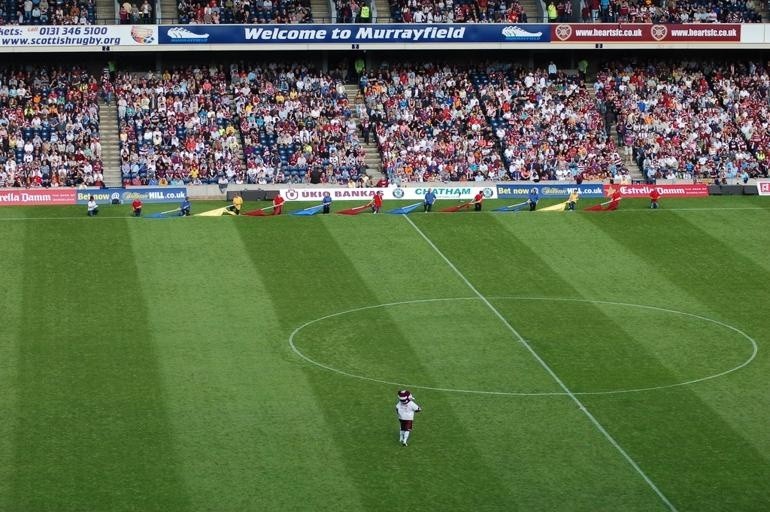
[399,439,409,448]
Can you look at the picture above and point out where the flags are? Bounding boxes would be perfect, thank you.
[583,201,618,211]
[537,201,567,211]
[335,204,371,215]
[384,201,424,214]
[193,207,237,216]
[490,201,528,212]
[242,206,281,216]
[143,212,178,218]
[287,205,323,216]
[437,201,473,212]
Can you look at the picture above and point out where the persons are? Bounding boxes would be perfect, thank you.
[229,193,243,215]
[132,197,143,216]
[322,192,332,215]
[471,190,484,211]
[649,187,661,208]
[528,189,539,211]
[0,0,770,189]
[372,190,383,214]
[395,389,422,447]
[272,193,285,214]
[87,196,99,217]
[568,190,579,210]
[611,189,620,210]
[180,197,191,217]
[424,190,436,213]
